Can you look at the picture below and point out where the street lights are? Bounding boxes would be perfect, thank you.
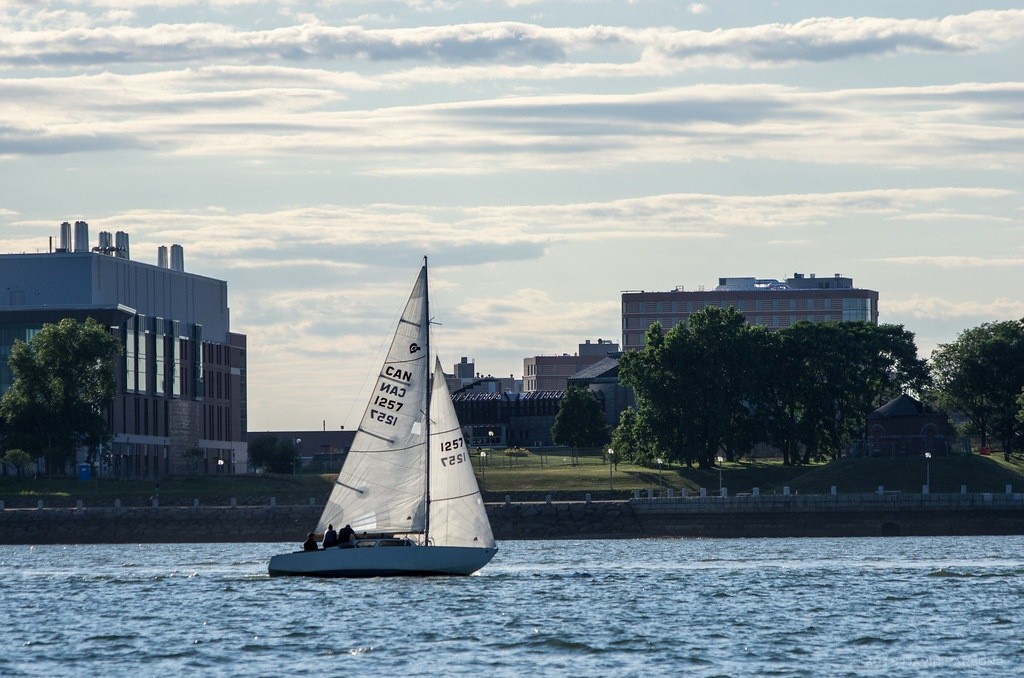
[217,459,225,503]
[489,430,494,461]
[480,452,486,491]
[608,448,614,491]
[657,458,664,497]
[717,456,724,496]
[925,452,932,495]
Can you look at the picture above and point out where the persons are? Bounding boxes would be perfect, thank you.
[322,524,337,549]
[338,525,359,548]
[304,532,318,552]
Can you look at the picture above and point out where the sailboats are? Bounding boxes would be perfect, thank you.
[267,253,499,577]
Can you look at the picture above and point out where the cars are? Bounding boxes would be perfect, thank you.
[979,447,992,455]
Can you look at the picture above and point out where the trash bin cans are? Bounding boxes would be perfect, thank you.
[477,447,482,451]
[466,444,471,452]
[76,463,91,481]
[514,445,518,450]
